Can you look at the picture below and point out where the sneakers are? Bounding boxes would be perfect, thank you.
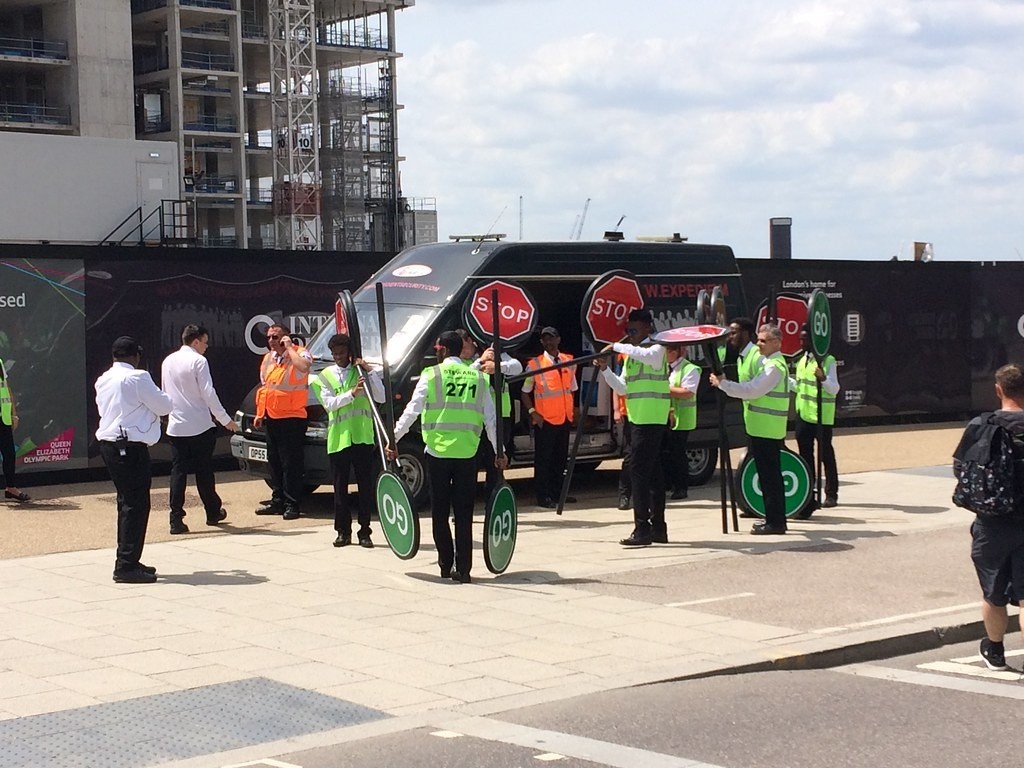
[979,638,1008,671]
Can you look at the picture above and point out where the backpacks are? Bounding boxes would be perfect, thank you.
[952,413,1022,522]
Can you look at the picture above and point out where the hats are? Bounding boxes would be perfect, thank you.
[542,327,560,336]
[112,336,143,358]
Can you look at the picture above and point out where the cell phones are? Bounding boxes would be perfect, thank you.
[281,335,289,347]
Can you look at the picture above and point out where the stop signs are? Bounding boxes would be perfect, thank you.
[586,274,644,345]
[335,298,350,338]
[651,325,727,344]
[715,297,726,348]
[470,280,536,341]
[754,296,810,358]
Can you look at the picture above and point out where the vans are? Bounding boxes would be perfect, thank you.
[229,231,748,514]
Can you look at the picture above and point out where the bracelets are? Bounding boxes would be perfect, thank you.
[528,407,536,413]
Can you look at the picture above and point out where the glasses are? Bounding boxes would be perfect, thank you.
[757,338,776,343]
[624,326,647,335]
[266,335,279,340]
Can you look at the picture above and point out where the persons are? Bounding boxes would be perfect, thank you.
[308,334,386,549]
[94,337,174,583]
[253,326,314,521]
[384,330,509,580]
[788,325,841,508]
[952,366,1023,672]
[730,318,799,519]
[449,329,523,522]
[613,352,633,508]
[709,323,789,535]
[0,355,30,501]
[664,341,702,498]
[592,310,669,546]
[162,326,239,534]
[521,326,578,506]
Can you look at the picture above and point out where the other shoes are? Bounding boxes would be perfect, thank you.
[283,508,300,519]
[452,572,472,583]
[618,498,629,509]
[255,504,284,516]
[620,532,651,545]
[170,524,188,534]
[207,508,227,525]
[566,497,576,503]
[653,534,667,542]
[359,536,373,547]
[672,491,688,500]
[5,491,30,501]
[441,570,449,578]
[823,496,838,507]
[333,530,352,546]
[113,562,157,583]
[540,498,556,508]
[751,520,788,535]
